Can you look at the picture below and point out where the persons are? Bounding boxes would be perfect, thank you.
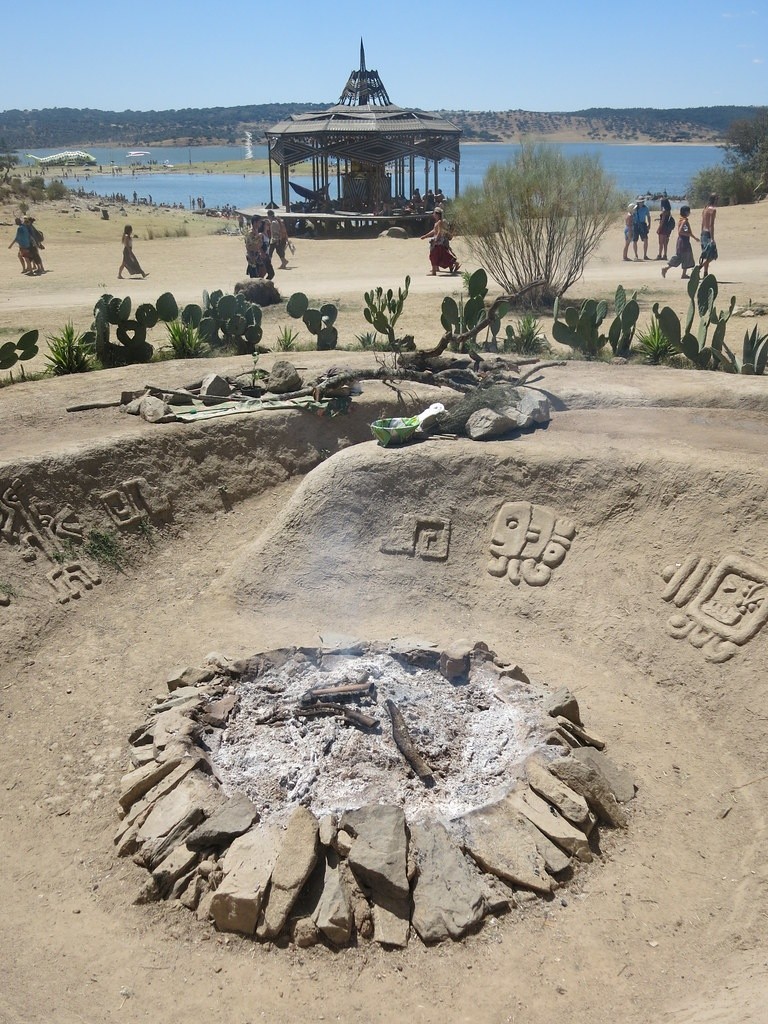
[622,196,651,263]
[117,224,150,280]
[661,205,701,280]
[16,161,252,230]
[698,193,720,279]
[330,160,334,168]
[245,210,289,281]
[421,207,462,276]
[8,217,44,277]
[654,198,673,260]
[372,189,447,216]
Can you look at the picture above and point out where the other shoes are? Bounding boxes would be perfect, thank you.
[622,258,632,261]
[278,261,288,269]
[450,264,456,272]
[654,255,662,261]
[644,256,652,260]
[117,276,124,279]
[662,268,667,278]
[143,272,150,278]
[21,267,45,276]
[426,273,436,276]
[634,256,639,262]
[661,255,667,260]
[454,263,462,272]
[681,274,690,279]
[430,268,439,272]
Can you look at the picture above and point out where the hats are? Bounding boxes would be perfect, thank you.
[628,203,638,211]
[636,195,645,202]
[434,207,443,213]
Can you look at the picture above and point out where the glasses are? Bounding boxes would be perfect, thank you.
[25,220,31,223]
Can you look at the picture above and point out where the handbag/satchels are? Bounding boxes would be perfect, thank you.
[638,222,649,234]
[665,215,675,234]
[699,241,718,265]
